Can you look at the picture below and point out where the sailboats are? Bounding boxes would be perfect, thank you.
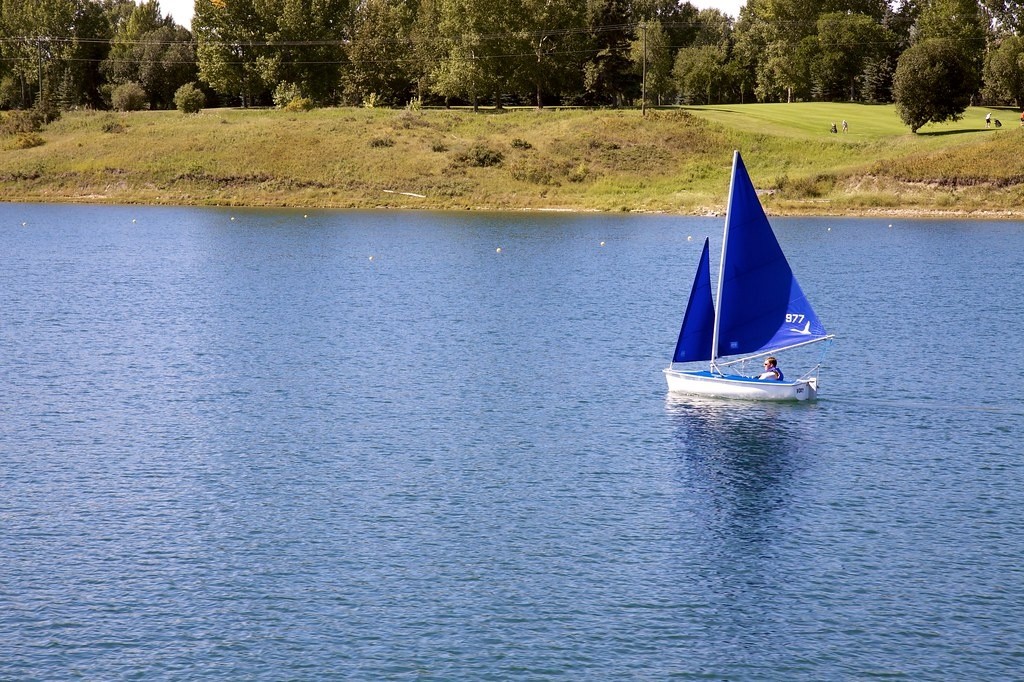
[663,153,835,406]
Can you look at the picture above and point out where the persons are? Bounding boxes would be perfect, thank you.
[985,111,1024,129]
[759,357,783,381]
[842,120,848,133]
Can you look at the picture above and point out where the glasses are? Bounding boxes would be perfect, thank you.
[764,363,769,366]
